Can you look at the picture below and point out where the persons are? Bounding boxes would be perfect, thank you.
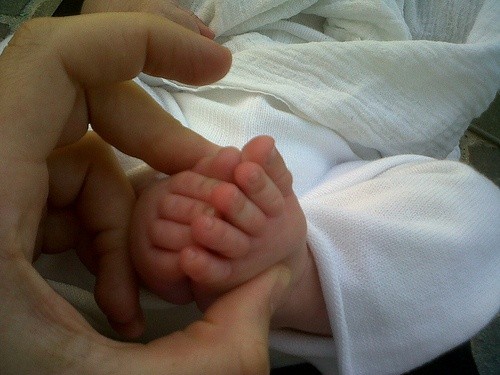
[1,11,291,375]
[74,0,500,375]
